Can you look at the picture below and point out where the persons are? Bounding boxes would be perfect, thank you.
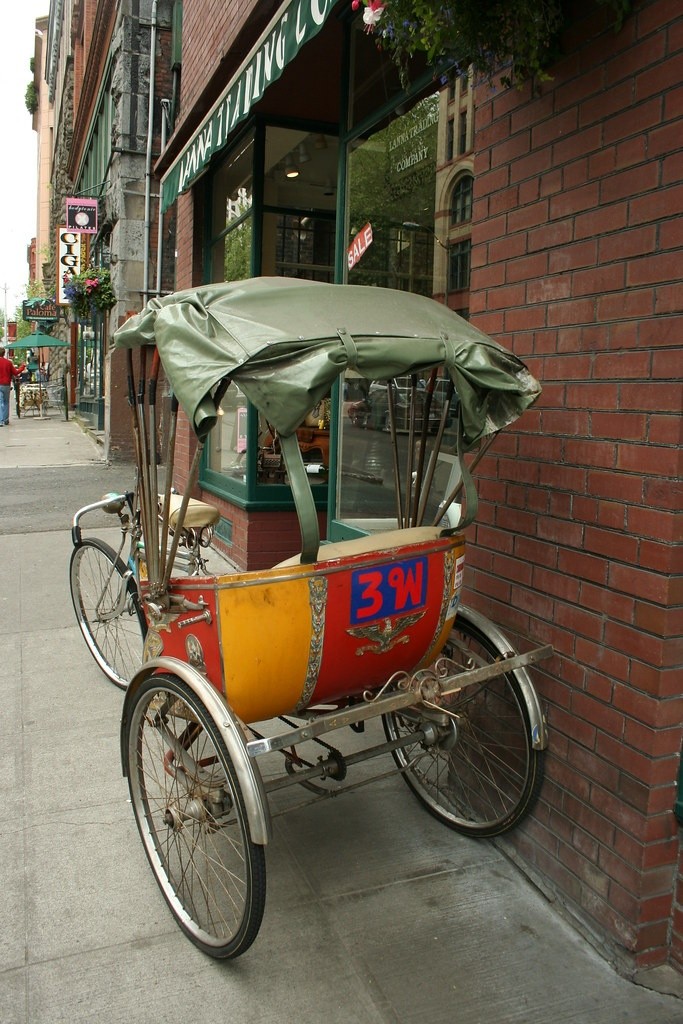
[0,347,47,427]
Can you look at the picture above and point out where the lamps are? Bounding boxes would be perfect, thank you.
[284,154,302,179]
[291,209,314,241]
[323,179,335,196]
[312,134,328,151]
[299,142,312,165]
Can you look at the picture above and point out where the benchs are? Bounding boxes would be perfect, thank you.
[139,526,466,723]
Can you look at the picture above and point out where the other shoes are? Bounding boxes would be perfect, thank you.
[4,420,9,424]
[0,424,4,427]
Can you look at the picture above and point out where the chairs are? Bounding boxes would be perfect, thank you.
[43,386,65,418]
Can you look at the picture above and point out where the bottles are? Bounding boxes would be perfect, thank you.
[304,464,329,474]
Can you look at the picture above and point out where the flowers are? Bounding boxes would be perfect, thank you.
[62,265,117,321]
[351,0,638,94]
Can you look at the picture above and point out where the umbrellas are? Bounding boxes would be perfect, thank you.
[3,331,71,418]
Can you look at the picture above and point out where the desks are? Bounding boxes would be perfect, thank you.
[24,387,49,418]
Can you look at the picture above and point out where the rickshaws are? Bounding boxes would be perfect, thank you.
[69,277,554,961]
